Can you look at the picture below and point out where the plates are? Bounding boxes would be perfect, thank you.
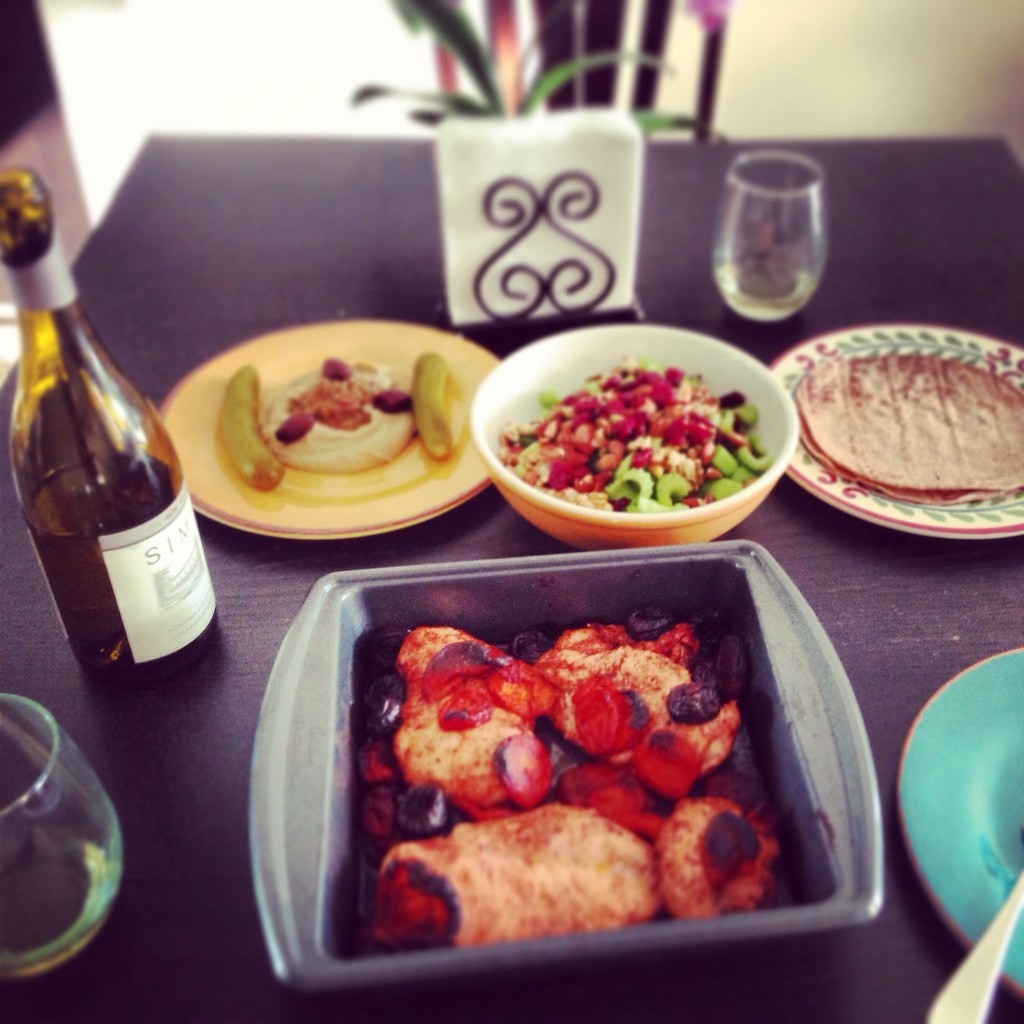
[898,646,1024,1001]
[767,322,1024,540]
[160,319,502,541]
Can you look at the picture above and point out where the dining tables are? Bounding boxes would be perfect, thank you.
[0,134,1024,1024]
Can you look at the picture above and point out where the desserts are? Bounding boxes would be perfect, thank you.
[261,357,411,476]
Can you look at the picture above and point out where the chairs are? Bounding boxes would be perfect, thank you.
[433,0,728,140]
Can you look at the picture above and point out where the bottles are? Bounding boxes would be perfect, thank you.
[0,169,221,684]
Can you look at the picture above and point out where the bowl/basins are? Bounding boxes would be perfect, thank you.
[469,322,799,552]
[247,542,885,1024]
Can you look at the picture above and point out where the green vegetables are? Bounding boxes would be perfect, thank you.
[541,360,772,513]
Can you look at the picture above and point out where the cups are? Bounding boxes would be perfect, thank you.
[711,149,828,325]
[0,691,122,980]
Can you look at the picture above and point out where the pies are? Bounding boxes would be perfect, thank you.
[793,356,1023,506]
